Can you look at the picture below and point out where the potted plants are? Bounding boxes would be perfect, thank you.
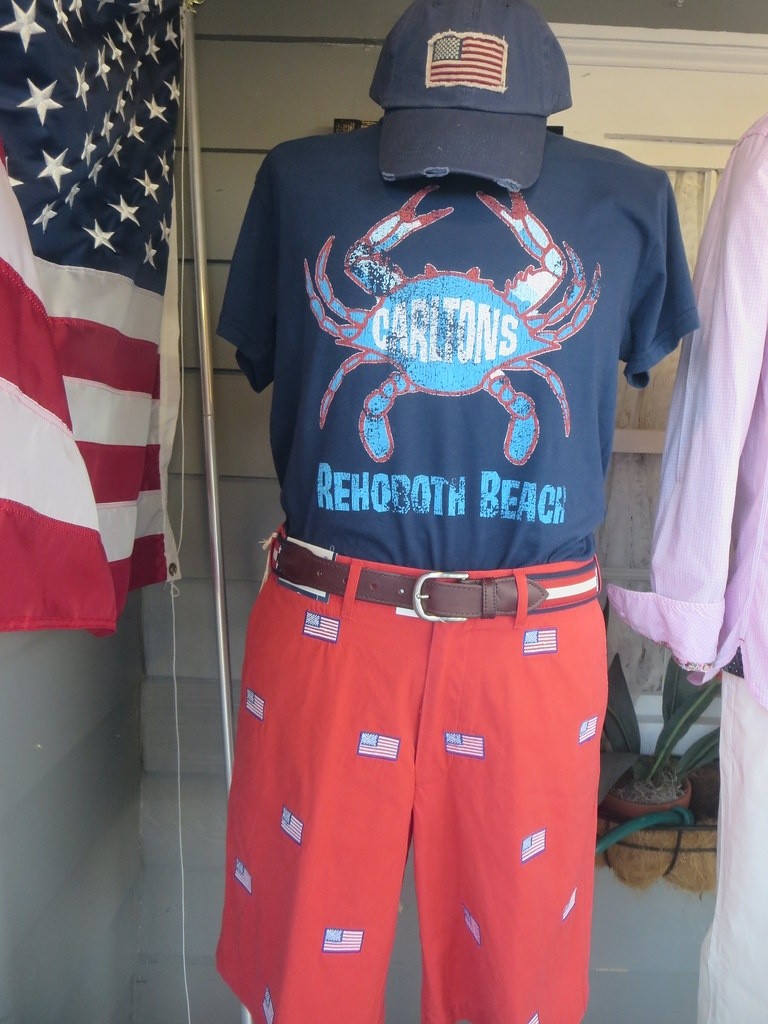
[592,652,720,893]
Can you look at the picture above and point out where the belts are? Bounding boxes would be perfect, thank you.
[271,536,600,623]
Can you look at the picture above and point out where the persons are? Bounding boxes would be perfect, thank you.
[215,0,703,1024]
[605,114,768,1023]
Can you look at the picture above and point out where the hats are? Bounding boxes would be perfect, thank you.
[369,0,572,192]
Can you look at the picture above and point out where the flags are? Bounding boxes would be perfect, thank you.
[0,0,186,639]
